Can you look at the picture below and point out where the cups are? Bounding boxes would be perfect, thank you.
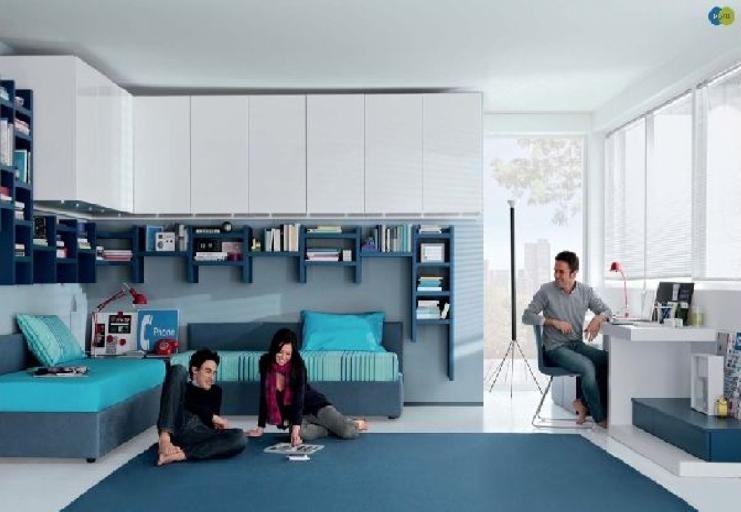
[691,303,705,326]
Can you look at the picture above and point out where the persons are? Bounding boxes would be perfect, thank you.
[156,350,248,466]
[244,327,369,446]
[522,251,612,429]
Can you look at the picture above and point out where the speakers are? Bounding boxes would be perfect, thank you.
[155,232,176,251]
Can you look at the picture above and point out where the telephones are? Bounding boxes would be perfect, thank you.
[156,339,178,356]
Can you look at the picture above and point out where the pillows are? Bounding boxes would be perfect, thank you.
[18,311,85,367]
[302,310,386,352]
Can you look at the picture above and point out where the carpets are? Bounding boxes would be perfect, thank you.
[60,431,701,511]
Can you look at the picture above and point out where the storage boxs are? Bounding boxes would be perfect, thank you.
[632,394,736,461]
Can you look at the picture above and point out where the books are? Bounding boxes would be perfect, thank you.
[263,442,324,455]
[102,249,132,260]
[194,250,228,262]
[306,247,341,261]
[417,276,443,291]
[32,367,89,377]
[416,300,450,320]
[264,223,299,253]
[374,223,413,253]
[307,225,343,234]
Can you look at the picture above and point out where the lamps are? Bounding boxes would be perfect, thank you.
[607,261,633,325]
[91,283,149,310]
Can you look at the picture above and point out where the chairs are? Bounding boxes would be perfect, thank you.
[534,321,595,429]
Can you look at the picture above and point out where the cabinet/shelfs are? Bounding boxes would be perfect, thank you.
[422,94,482,218]
[0,53,135,215]
[365,94,423,222]
[135,96,190,219]
[191,96,248,218]
[0,78,34,288]
[307,94,364,219]
[248,96,307,217]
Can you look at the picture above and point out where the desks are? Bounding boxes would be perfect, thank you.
[581,311,720,407]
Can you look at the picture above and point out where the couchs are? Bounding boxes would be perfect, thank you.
[0,331,168,463]
[170,322,403,420]
